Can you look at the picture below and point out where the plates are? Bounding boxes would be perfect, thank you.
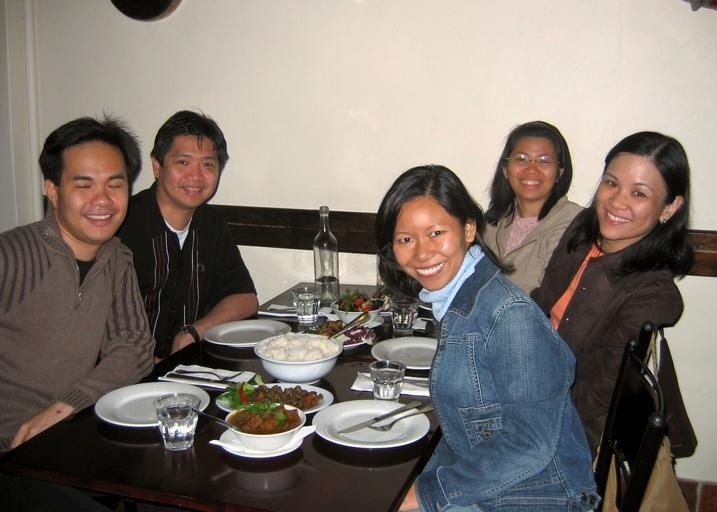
[312,399,430,450]
[215,383,335,414]
[369,316,385,331]
[370,336,438,371]
[344,334,377,348]
[203,319,291,348]
[220,429,306,459]
[93,380,211,428]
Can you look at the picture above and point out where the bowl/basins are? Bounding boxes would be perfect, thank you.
[224,403,307,450]
[330,298,382,324]
[254,333,344,385]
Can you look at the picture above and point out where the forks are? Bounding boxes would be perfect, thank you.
[362,402,434,431]
[175,367,245,381]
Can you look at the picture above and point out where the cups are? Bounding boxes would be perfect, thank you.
[153,391,201,451]
[291,286,322,327]
[389,295,419,337]
[369,360,405,400]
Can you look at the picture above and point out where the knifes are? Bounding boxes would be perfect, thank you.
[338,400,422,433]
[167,373,237,385]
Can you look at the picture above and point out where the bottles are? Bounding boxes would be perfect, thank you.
[313,205,340,307]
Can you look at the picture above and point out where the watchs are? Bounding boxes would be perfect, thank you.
[180,321,200,343]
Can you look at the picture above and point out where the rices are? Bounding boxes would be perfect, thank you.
[260,332,340,363]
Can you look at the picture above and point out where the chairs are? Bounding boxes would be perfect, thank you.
[597,321,664,510]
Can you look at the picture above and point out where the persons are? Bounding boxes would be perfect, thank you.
[528,131,694,464]
[374,163,603,512]
[480,120,589,299]
[111,109,260,364]
[0,114,157,457]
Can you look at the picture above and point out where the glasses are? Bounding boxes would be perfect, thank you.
[502,154,561,171]
